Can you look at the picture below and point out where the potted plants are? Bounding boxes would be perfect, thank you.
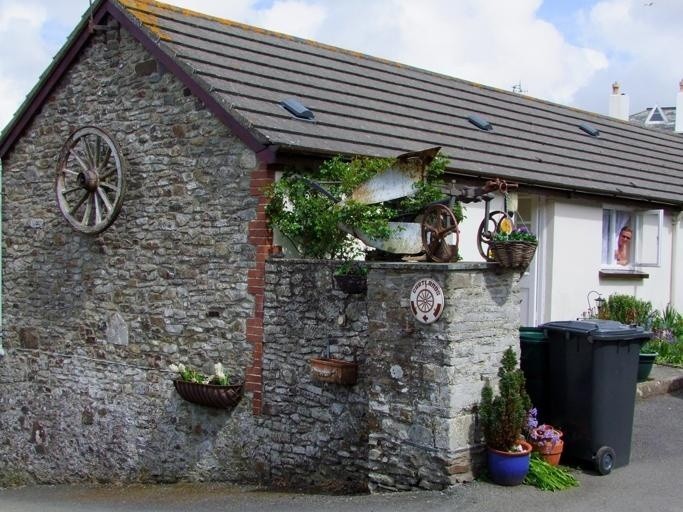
[332,256,369,293]
[596,294,659,382]
[478,345,532,487]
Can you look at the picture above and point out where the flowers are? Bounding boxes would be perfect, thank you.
[526,408,560,456]
[169,362,234,385]
[488,217,536,249]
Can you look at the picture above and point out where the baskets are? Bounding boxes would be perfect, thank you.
[487,209,539,270]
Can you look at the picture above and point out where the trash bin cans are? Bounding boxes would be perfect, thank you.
[520,319,652,475]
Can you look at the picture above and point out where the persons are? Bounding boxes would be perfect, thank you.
[615,227,632,265]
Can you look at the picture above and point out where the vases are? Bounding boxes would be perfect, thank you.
[308,359,358,386]
[531,424,565,466]
[172,377,243,410]
[488,241,537,270]
